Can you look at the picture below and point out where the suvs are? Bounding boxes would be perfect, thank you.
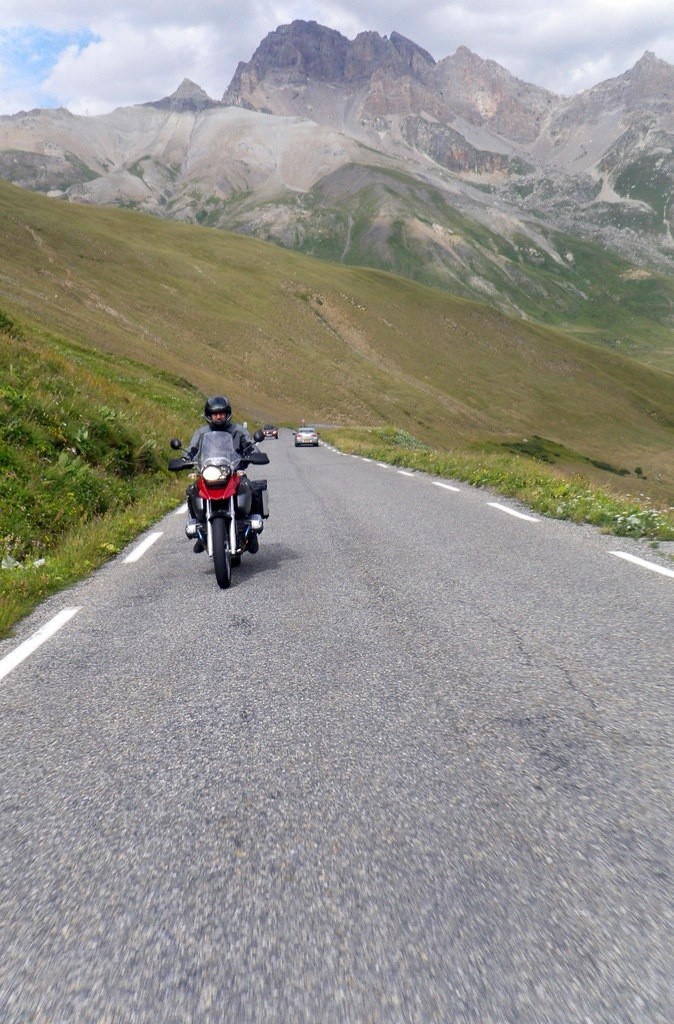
[261,424,278,439]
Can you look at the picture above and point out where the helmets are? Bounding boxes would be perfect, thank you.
[205,397,233,426]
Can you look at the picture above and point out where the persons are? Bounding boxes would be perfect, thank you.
[181,395,260,554]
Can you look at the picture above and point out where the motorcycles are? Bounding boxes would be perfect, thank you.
[168,431,269,589]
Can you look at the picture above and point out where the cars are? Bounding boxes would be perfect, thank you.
[293,428,321,447]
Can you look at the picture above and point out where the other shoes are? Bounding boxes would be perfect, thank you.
[194,540,204,554]
[248,533,259,554]
